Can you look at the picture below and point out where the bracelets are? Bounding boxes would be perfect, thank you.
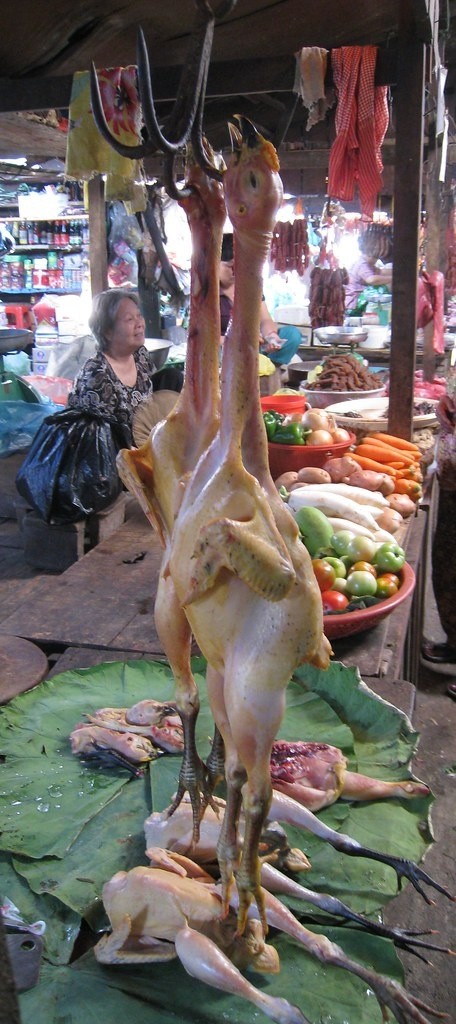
[268,330,277,335]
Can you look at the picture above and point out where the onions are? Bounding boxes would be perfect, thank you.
[281,408,350,447]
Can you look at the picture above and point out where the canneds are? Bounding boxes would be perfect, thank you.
[47,252,57,270]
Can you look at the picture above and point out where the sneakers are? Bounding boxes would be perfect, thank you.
[448,684,456,695]
[420,642,456,676]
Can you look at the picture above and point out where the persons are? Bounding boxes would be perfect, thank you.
[343,231,393,312]
[67,290,184,449]
[218,233,302,368]
[420,360,456,698]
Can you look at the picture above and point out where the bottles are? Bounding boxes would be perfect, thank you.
[0,219,91,291]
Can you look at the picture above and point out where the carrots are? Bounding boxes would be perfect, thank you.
[343,432,424,501]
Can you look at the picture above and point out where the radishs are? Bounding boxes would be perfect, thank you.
[279,481,395,543]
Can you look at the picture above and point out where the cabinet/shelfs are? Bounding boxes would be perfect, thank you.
[0,213,90,294]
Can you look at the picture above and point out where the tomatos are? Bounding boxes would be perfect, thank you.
[312,530,405,613]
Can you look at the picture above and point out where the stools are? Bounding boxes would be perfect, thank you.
[5,306,32,331]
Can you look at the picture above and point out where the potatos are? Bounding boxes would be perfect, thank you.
[274,456,416,517]
[374,507,403,533]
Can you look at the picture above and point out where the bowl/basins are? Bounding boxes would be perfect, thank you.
[143,336,173,370]
[319,561,416,643]
[266,432,359,485]
[296,384,386,409]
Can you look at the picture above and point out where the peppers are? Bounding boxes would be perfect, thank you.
[262,409,313,446]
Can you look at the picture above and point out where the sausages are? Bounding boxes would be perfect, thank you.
[272,219,309,277]
[307,266,350,328]
[358,222,393,259]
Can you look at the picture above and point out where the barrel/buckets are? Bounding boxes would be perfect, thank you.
[260,393,306,418]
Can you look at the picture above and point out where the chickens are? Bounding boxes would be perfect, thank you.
[91,736,455,1024]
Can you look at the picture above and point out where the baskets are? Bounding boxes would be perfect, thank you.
[323,556,415,640]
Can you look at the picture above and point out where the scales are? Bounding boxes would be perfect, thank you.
[314,326,371,370]
[370,294,393,326]
[0,328,52,407]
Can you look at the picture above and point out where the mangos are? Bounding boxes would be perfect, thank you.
[293,506,331,551]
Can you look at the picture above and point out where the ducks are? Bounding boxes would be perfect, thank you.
[112,108,337,946]
